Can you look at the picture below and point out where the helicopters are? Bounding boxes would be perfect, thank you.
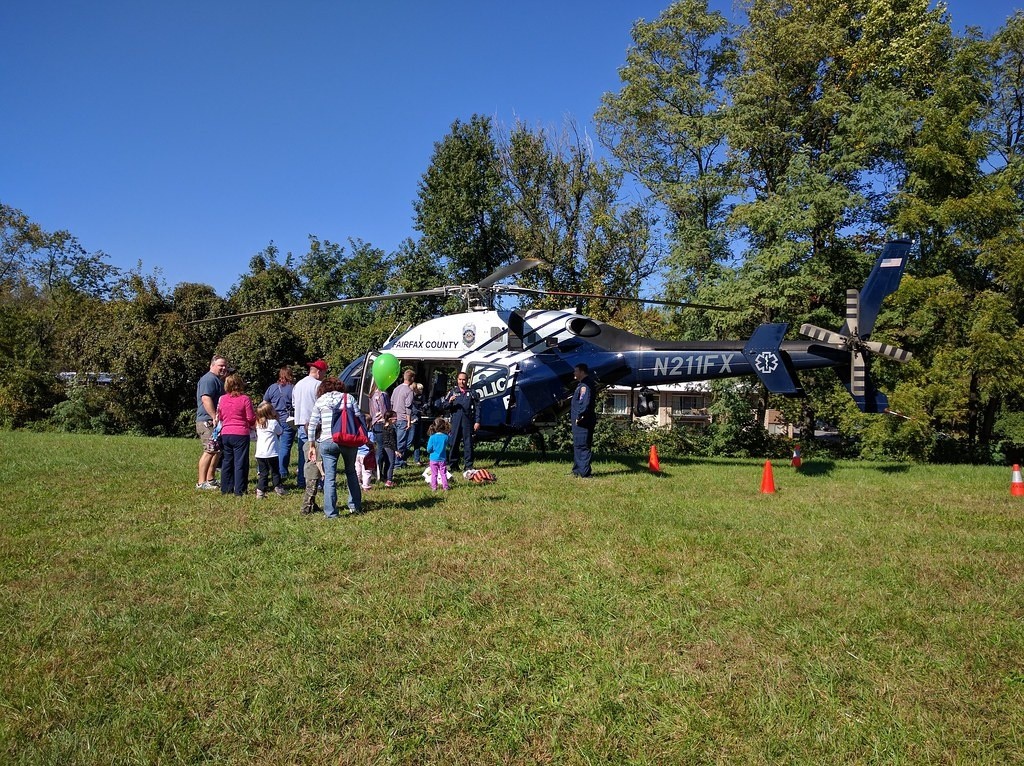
[184,239,917,479]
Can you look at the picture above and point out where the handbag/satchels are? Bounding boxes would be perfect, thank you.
[331,392,368,447]
[473,470,497,484]
[286,411,299,430]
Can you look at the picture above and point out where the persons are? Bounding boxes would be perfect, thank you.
[194,355,424,517]
[570,363,597,477]
[427,417,452,492]
[443,372,481,472]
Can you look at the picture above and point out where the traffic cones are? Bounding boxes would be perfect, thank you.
[647,445,661,474]
[760,460,779,492]
[791,444,803,467]
[1010,465,1024,496]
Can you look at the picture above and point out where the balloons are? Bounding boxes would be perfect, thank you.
[372,353,400,392]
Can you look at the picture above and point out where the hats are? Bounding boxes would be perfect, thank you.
[307,360,328,372]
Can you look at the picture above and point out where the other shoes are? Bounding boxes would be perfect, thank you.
[196,479,221,490]
[275,487,291,496]
[257,489,268,500]
[385,480,397,488]
